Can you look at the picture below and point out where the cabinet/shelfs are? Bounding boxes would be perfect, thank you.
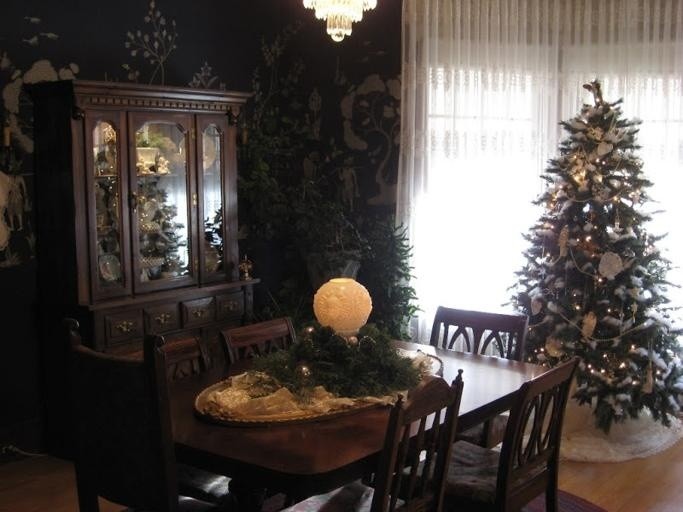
[27,78,263,452]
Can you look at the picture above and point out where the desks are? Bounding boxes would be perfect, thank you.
[166,333,548,512]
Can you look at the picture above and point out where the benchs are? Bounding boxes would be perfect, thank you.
[389,350,581,512]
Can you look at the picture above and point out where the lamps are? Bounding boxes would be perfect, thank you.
[312,275,373,347]
[301,0,379,44]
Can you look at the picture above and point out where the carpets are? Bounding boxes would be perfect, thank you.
[114,460,608,511]
[491,383,682,465]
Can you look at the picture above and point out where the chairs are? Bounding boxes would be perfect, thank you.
[115,332,234,503]
[216,313,301,372]
[55,316,233,512]
[279,365,465,512]
[427,304,532,450]
